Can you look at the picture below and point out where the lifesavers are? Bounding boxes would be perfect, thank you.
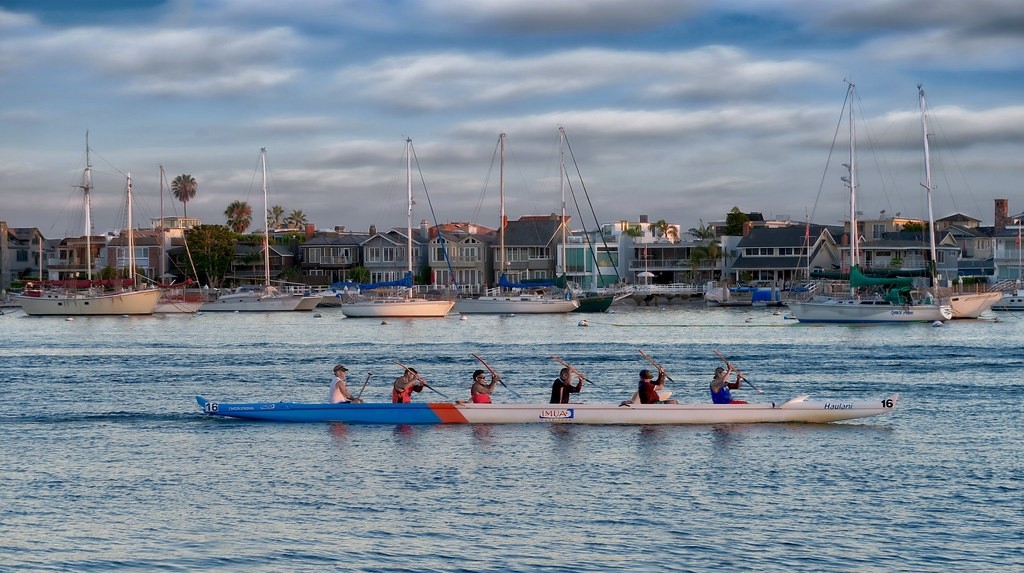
[26,282,33,288]
[564,292,572,301]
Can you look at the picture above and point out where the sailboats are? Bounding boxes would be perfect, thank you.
[989,219,1024,311]
[12,127,209,315]
[478,127,635,313]
[426,132,579,314]
[899,84,1004,320]
[195,146,322,312]
[341,133,456,318]
[780,81,952,323]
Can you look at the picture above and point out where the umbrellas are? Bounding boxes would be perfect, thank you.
[159,273,178,285]
[637,272,654,285]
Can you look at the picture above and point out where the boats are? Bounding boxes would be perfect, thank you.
[310,287,359,308]
[194,391,900,424]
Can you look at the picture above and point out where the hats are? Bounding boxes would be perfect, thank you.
[639,369,654,379]
[713,368,725,379]
[333,365,348,371]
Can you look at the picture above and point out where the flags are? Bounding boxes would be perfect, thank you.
[643,247,647,256]
[1016,235,1021,248]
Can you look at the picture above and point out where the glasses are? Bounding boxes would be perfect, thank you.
[477,377,485,379]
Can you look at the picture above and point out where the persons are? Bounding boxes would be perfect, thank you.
[471,370,500,405]
[639,366,678,404]
[329,365,363,404]
[549,364,585,404]
[1012,285,1019,296]
[710,361,748,404]
[393,368,427,403]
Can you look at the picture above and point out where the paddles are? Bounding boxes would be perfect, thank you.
[550,353,608,391]
[394,360,449,399]
[356,372,372,399]
[712,348,764,393]
[637,348,675,383]
[471,353,523,399]
